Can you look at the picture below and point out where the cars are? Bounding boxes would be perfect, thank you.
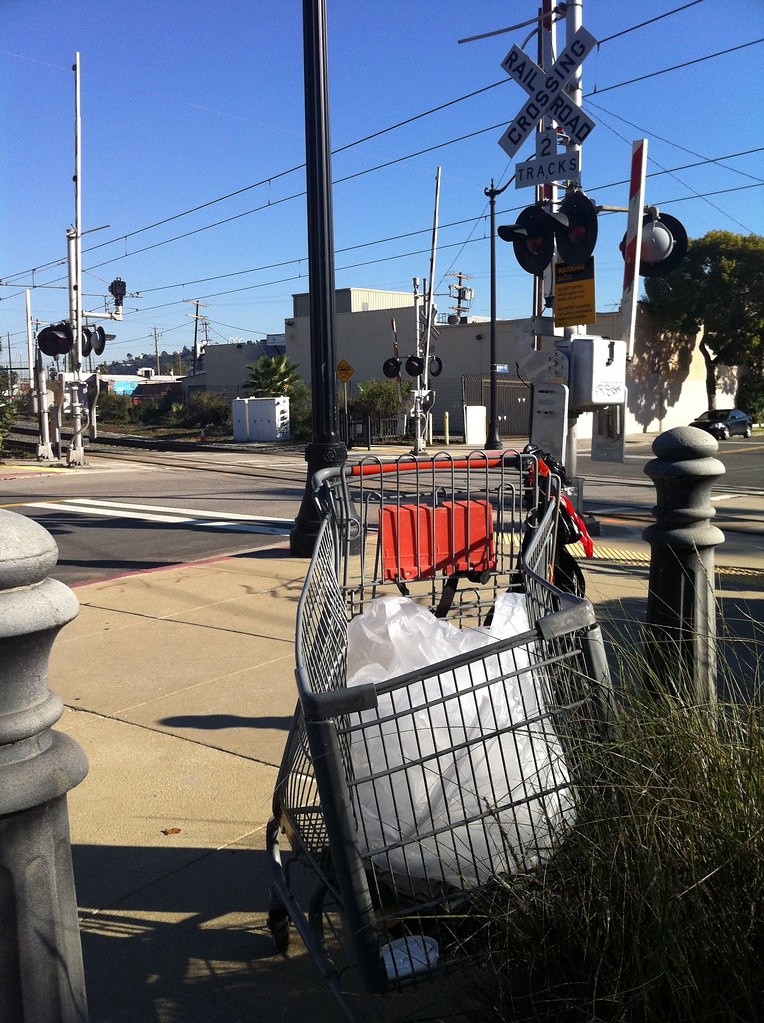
[688,409,752,440]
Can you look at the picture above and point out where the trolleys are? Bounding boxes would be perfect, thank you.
[261,443,618,1023]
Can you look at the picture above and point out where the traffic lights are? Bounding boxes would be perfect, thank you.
[383,357,424,378]
[497,192,598,275]
[82,326,116,357]
